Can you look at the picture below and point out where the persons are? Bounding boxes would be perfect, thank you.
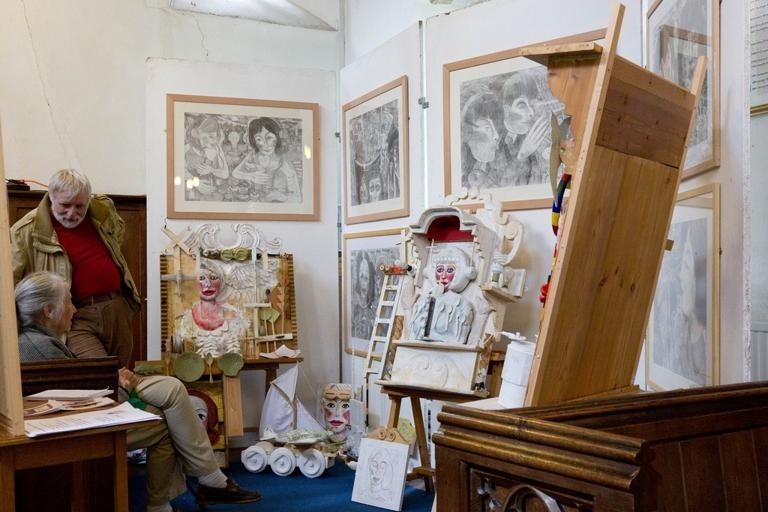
[186,391,221,447]
[10,169,141,372]
[231,117,303,205]
[554,116,576,189]
[350,251,379,339]
[357,446,395,505]
[460,95,508,189]
[187,118,229,191]
[172,261,254,358]
[495,73,551,187]
[13,272,263,512]
[318,385,356,443]
[367,171,385,202]
[408,247,478,345]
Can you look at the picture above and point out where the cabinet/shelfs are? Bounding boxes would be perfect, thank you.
[9,192,147,372]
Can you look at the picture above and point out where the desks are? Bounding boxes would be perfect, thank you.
[0,395,156,511]
[433,374,767,507]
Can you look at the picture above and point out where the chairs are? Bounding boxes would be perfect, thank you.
[21,355,118,511]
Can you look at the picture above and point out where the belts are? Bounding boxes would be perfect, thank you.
[76,287,125,310]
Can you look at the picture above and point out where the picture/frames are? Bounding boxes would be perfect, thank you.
[344,231,405,359]
[647,185,717,394]
[648,0,720,176]
[661,28,709,147]
[342,75,410,225]
[166,93,320,222]
[444,26,605,209]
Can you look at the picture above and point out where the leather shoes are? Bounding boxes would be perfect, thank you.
[196,479,263,505]
